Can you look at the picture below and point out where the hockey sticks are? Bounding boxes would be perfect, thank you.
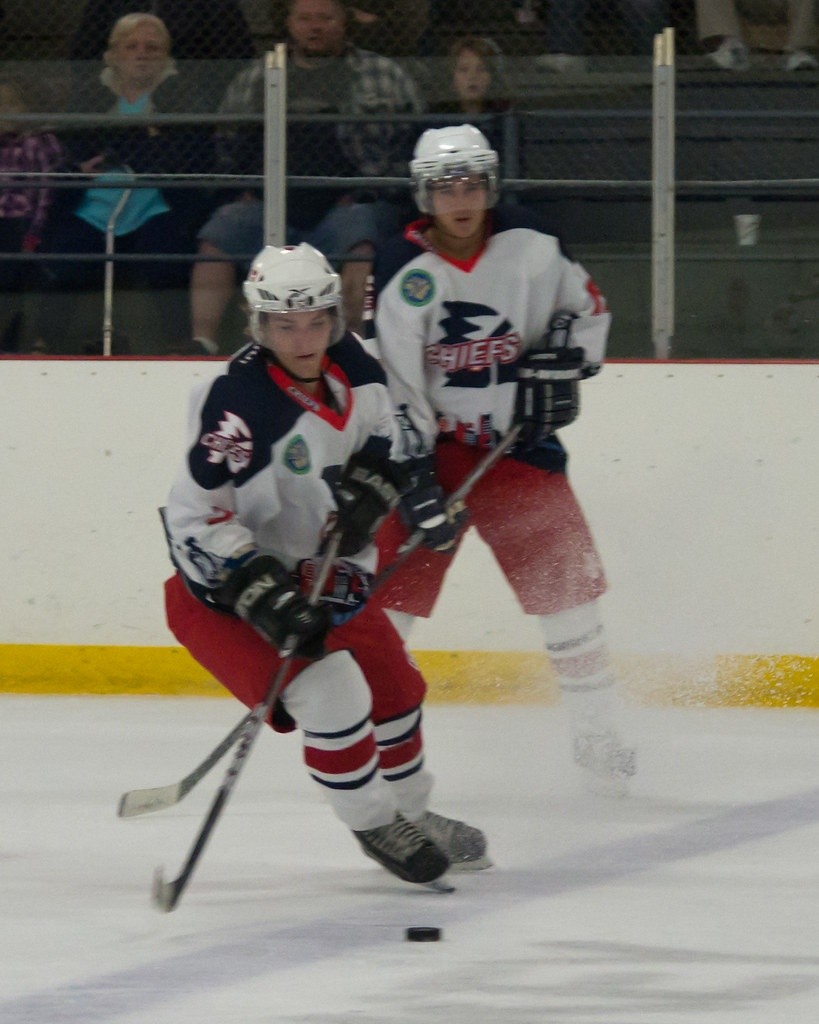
[120,422,523,816]
[155,526,345,912]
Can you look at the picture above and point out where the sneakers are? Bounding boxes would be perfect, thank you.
[351,809,498,895]
[564,711,641,780]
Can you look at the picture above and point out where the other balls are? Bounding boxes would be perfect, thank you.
[407,926,441,940]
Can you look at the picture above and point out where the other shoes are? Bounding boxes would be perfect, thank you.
[30,335,220,354]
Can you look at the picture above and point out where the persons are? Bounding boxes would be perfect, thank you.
[0,73,62,355]
[422,33,583,276]
[53,14,229,361]
[677,1,817,250]
[374,125,640,772]
[184,3,422,359]
[160,237,487,885]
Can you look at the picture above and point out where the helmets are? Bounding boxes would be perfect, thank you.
[412,122,500,215]
[246,239,348,353]
